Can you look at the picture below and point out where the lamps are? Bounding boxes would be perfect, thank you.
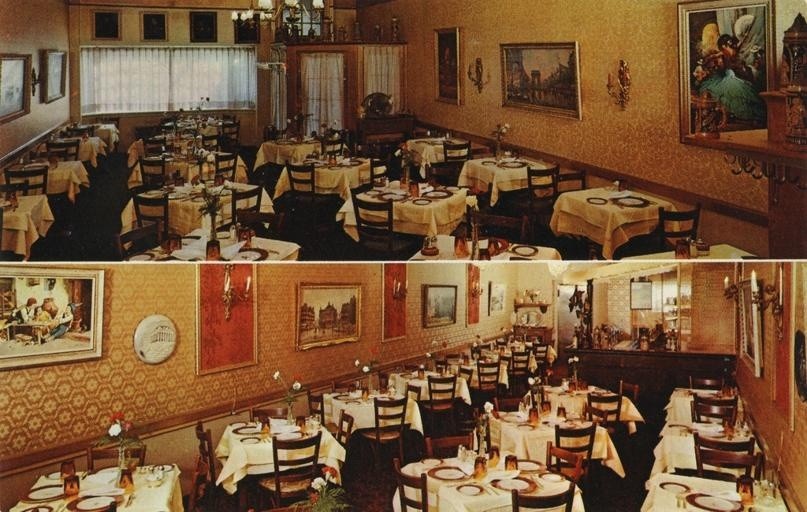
[744,259,789,331]
[392,274,411,302]
[722,270,742,300]
[604,57,634,115]
[30,63,41,98]
[228,0,342,34]
[466,56,486,93]
[470,280,486,303]
[219,264,255,322]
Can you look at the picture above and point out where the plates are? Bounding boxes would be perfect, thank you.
[151,463,174,473]
[667,422,725,439]
[45,471,61,480]
[331,388,394,408]
[229,421,306,445]
[144,139,648,260]
[422,456,569,497]
[20,483,123,511]
[658,480,743,511]
[500,411,575,432]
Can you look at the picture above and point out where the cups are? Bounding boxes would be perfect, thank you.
[63,475,80,498]
[418,368,424,380]
[261,420,270,433]
[542,400,551,416]
[558,406,566,419]
[296,415,306,433]
[61,461,75,479]
[529,408,538,425]
[736,478,753,505]
[119,469,134,491]
[674,239,711,259]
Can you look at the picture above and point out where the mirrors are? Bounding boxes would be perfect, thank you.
[521,311,544,328]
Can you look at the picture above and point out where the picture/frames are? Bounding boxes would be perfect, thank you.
[88,4,267,51]
[430,22,465,112]
[737,264,766,377]
[0,45,34,125]
[488,281,507,317]
[419,281,459,330]
[1,264,108,372]
[37,44,70,105]
[497,39,585,124]
[293,279,365,351]
[672,0,779,144]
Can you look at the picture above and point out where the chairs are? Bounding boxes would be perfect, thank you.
[1,326,796,512]
[1,118,762,259]
